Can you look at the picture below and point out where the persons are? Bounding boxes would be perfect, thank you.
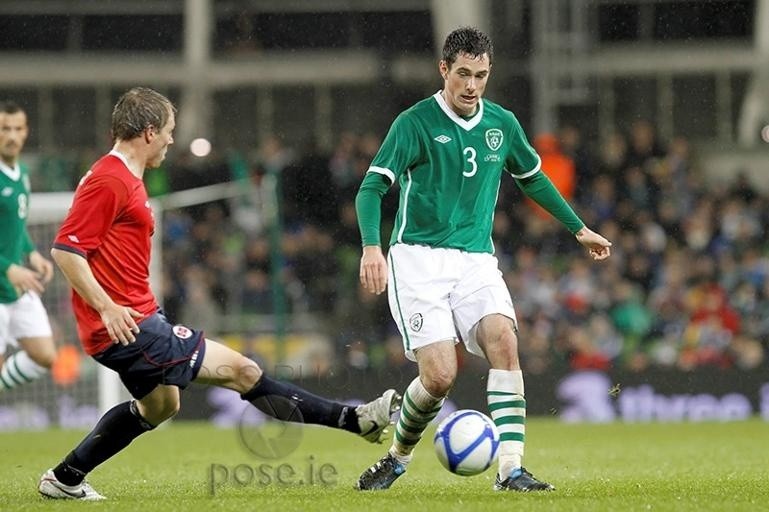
[352,25,614,492]
[27,118,769,373]
[36,83,402,501]
[0,97,58,392]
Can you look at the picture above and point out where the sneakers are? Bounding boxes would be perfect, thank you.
[352,389,402,445]
[37,468,108,501]
[358,452,407,490]
[494,466,555,494]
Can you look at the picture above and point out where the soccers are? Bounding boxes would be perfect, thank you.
[434,408,500,475]
